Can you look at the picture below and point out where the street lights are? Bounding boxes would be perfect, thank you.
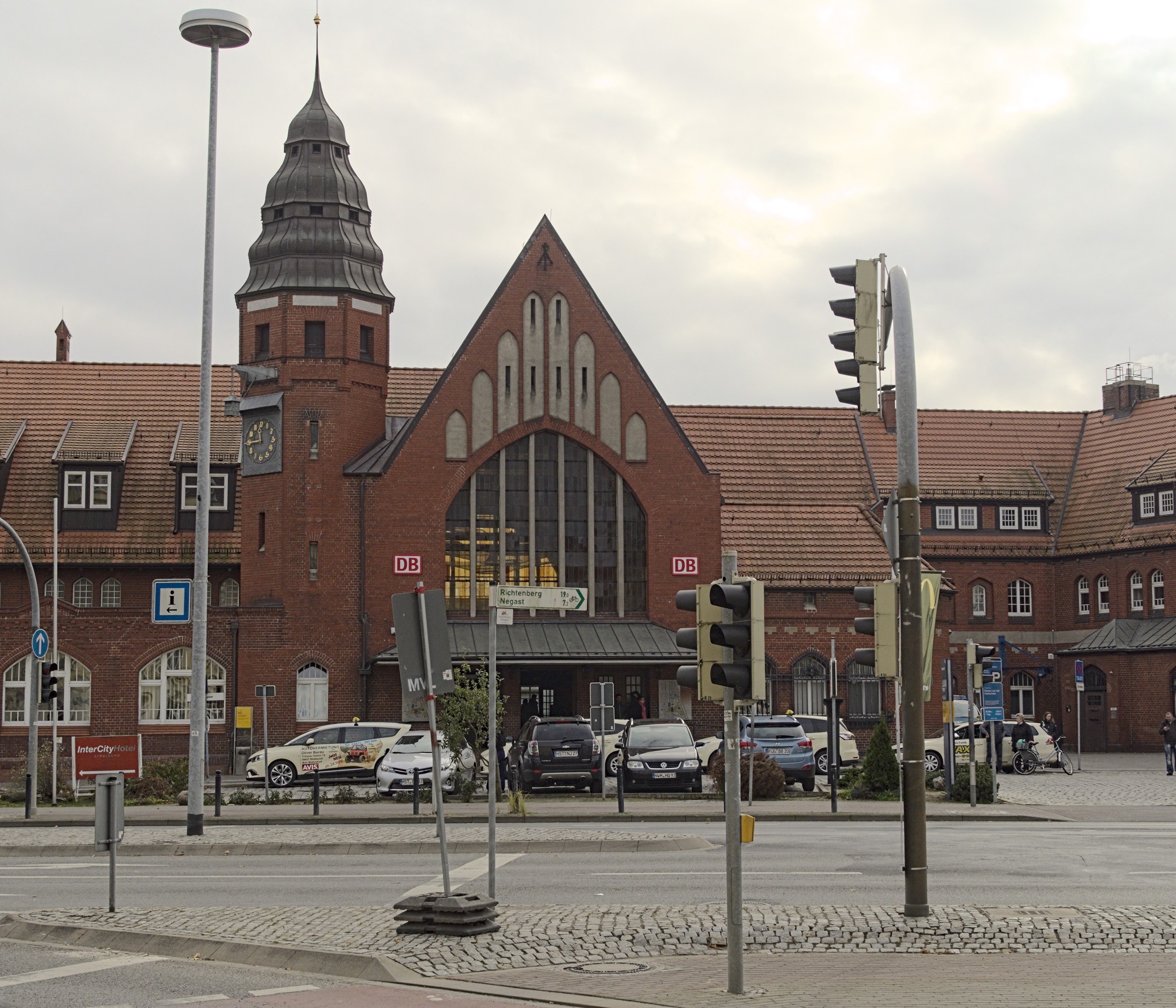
[168,6,260,835]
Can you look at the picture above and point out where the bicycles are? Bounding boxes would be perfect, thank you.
[1012,735,1074,775]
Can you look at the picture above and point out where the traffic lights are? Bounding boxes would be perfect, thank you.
[675,576,764,708]
[831,257,885,416]
[853,579,900,682]
[966,643,995,664]
[38,661,58,704]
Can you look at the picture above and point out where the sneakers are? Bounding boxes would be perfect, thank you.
[1023,770,1029,773]
[997,769,1006,774]
[1167,773,1172,775]
[1012,770,1018,774]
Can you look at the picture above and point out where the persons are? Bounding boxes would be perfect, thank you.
[527,694,539,718]
[630,691,641,701]
[1159,711,1176,776]
[979,721,1006,773]
[521,698,528,728]
[613,693,626,719]
[47,702,62,721]
[1040,711,1059,767]
[1011,713,1035,775]
[625,696,642,720]
[758,700,763,714]
[637,696,647,720]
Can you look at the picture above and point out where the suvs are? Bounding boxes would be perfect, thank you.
[953,695,982,723]
[479,709,859,790]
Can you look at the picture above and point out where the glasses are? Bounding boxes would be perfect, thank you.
[1046,716,1052,717]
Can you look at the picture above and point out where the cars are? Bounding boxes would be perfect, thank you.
[890,719,1058,772]
[247,717,478,795]
